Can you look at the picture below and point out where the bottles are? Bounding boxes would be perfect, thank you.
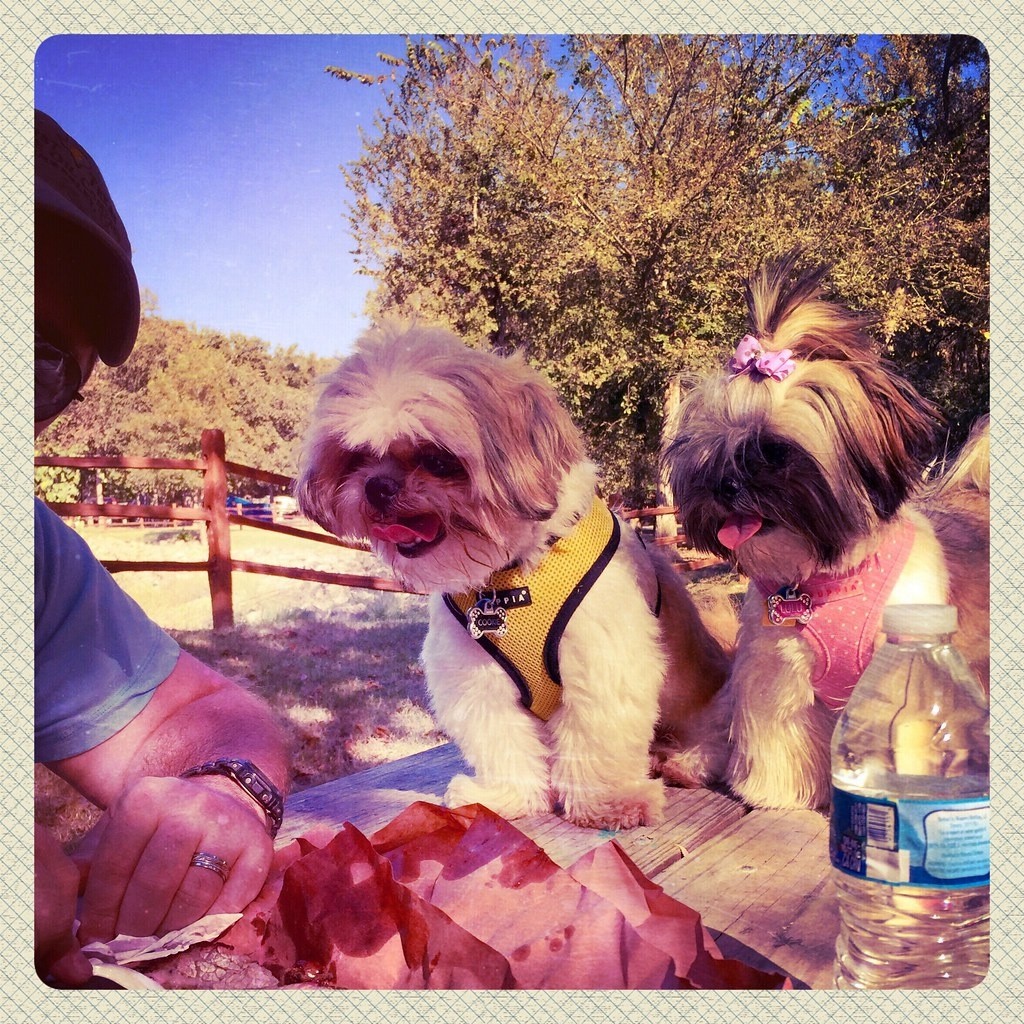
[827,604,989,990]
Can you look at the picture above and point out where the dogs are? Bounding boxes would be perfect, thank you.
[293,302,741,831]
[661,246,990,810]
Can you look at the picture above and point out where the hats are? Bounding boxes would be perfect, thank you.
[36,109,140,367]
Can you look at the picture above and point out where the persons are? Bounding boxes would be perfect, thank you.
[35,107,292,991]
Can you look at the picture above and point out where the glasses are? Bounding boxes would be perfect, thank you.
[35,333,84,422]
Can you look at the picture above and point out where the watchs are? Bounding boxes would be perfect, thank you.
[176,755,287,835]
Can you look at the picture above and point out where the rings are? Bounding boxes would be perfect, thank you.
[189,852,230,882]
